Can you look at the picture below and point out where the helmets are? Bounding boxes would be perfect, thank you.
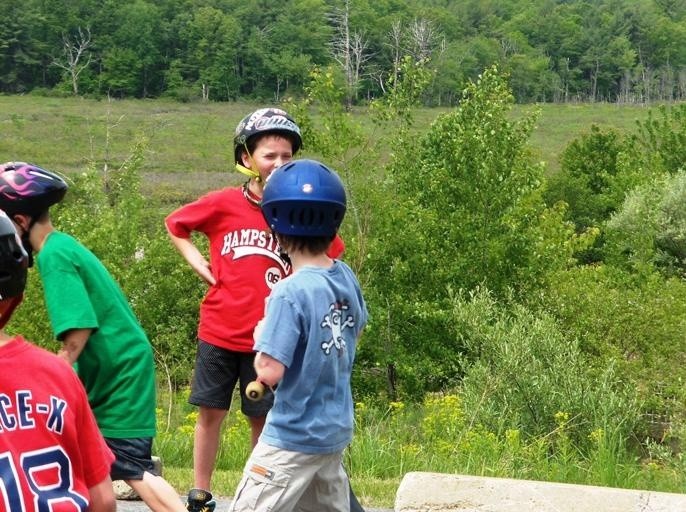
[261,158,347,242]
[0,210,29,330]
[234,108,302,165]
[0,162,69,218]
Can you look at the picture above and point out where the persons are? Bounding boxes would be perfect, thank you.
[0,158,189,512]
[225,157,371,512]
[0,209,119,512]
[161,108,346,511]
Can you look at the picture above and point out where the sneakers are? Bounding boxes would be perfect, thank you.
[183,488,216,512]
